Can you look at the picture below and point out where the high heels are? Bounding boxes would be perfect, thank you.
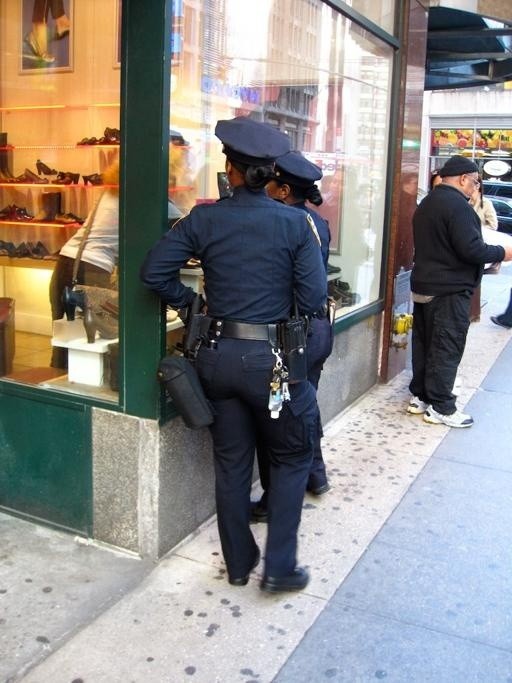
[24,159,105,185]
[61,286,87,321]
[84,310,118,343]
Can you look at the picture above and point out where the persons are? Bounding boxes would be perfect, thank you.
[431,169,481,323]
[490,289,512,328]
[465,175,499,233]
[47,137,204,375]
[403,154,512,429]
[244,148,331,526]
[141,115,330,594]
[401,172,419,274]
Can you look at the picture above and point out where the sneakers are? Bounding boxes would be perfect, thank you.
[424,405,474,427]
[407,395,428,414]
[327,264,361,306]
[259,567,308,592]
[250,500,268,523]
[491,316,511,329]
[306,479,330,495]
[229,545,260,585]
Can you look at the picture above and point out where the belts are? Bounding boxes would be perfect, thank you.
[222,321,269,341]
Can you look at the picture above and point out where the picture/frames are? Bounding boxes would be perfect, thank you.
[17,1,73,75]
[113,1,178,69]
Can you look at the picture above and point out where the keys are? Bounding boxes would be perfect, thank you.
[265,348,293,420]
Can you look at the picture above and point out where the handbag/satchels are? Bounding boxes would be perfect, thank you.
[72,284,118,317]
[158,357,214,430]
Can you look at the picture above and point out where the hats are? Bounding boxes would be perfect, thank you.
[277,148,322,187]
[215,115,290,167]
[440,155,478,176]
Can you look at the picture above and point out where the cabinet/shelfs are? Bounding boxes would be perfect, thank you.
[1,141,120,262]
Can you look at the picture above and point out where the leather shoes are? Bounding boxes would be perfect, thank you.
[0,204,33,220]
[55,27,70,39]
[78,127,121,145]
[24,32,55,63]
[166,308,179,322]
[0,240,59,261]
[55,212,83,223]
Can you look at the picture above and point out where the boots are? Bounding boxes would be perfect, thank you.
[33,191,52,222]
[0,132,19,183]
